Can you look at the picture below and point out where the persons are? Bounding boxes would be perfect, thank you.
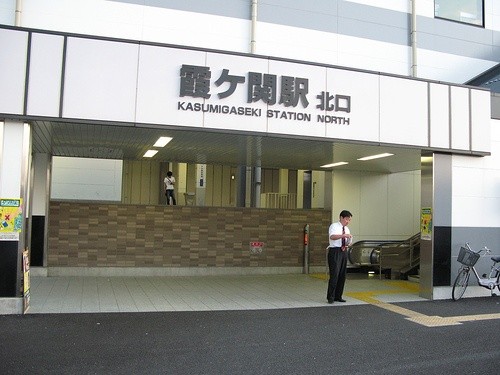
[164,171,177,205]
[326,210,352,305]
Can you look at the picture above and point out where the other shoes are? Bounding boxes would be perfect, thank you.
[328,297,334,304]
[335,298,346,302]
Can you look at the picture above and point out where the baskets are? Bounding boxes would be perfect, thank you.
[457,246,480,266]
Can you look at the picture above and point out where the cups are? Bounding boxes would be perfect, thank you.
[348,237,352,243]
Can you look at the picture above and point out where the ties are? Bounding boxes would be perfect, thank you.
[341,226,346,252]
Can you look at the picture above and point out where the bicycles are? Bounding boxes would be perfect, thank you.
[451,242,500,301]
